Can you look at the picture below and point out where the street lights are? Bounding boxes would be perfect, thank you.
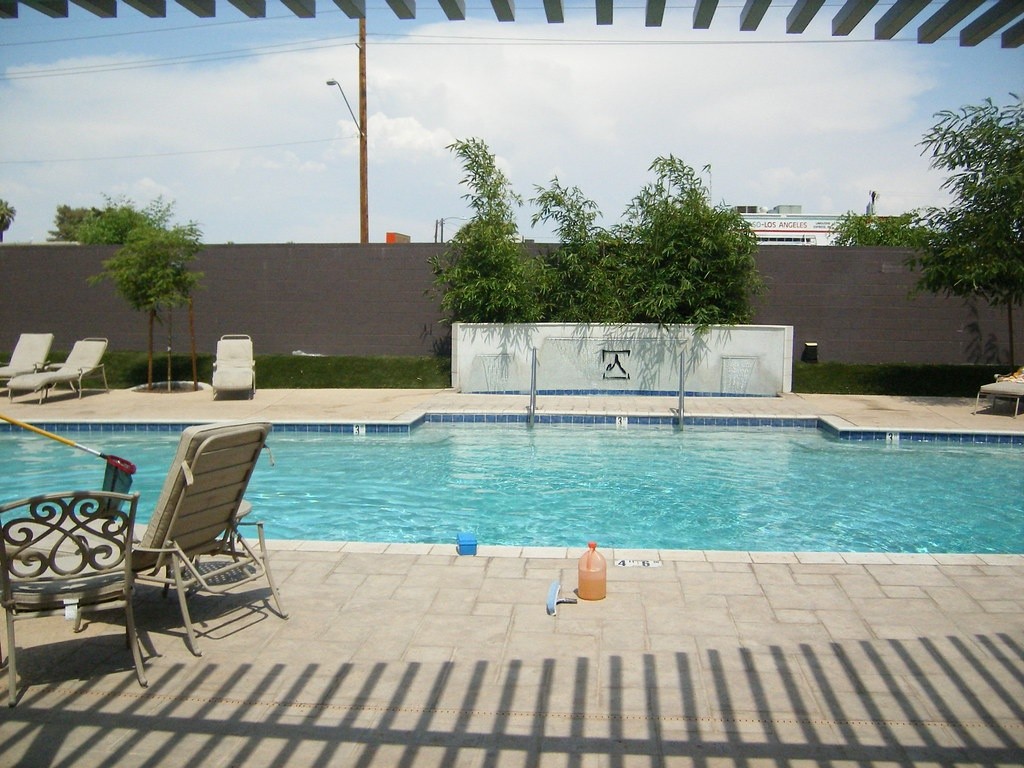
[434,216,468,243]
[327,80,370,244]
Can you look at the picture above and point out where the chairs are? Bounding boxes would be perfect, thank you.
[2,420,290,657]
[973,366,1024,419]
[0,489,149,707]
[0,333,55,399]
[6,338,110,406]
[212,334,256,400]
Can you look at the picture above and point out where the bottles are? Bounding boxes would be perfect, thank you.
[578,542,607,600]
